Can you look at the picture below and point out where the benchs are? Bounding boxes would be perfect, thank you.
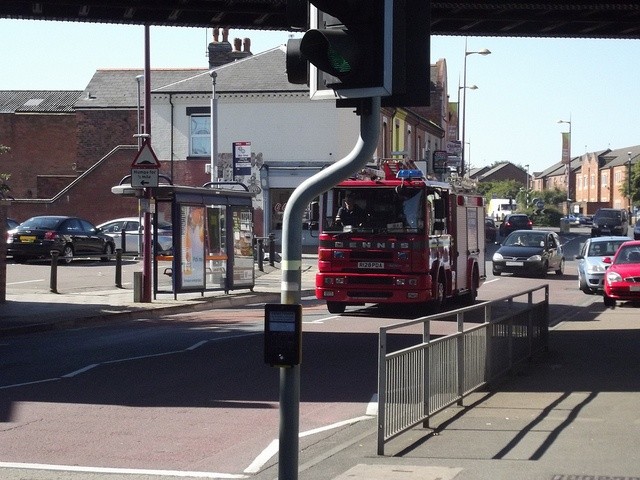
[165,266,226,291]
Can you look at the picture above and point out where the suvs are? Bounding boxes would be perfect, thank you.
[500,215,532,236]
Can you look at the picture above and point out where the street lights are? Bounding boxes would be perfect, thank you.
[525,165,529,209]
[210,71,222,283]
[628,152,632,225]
[558,120,571,218]
[136,75,144,257]
[458,85,478,140]
[462,49,491,176]
[467,142,470,179]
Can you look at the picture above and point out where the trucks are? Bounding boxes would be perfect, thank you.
[488,199,516,221]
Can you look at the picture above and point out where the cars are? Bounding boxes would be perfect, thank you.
[591,208,628,237]
[90,217,172,259]
[485,219,498,241]
[634,219,640,239]
[6,215,115,264]
[602,241,640,305]
[492,230,565,276]
[576,237,634,295]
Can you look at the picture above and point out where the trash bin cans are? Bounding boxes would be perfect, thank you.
[133,272,144,303]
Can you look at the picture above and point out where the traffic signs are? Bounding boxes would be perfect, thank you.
[131,169,159,187]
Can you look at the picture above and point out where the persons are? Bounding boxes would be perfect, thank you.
[592,244,608,255]
[335,191,366,226]
[514,236,528,246]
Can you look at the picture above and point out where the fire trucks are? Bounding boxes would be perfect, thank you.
[309,159,486,313]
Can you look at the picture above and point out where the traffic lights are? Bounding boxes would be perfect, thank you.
[300,0,393,100]
